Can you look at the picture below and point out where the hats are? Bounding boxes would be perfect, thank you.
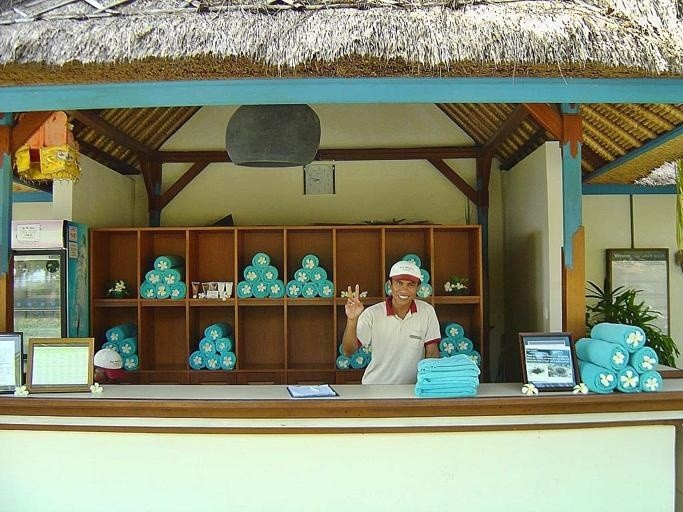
[388,260,422,284]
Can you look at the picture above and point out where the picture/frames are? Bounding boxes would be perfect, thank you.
[519,332,581,392]
[26,338,95,393]
[0,332,23,394]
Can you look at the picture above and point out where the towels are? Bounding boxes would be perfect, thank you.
[418,354,481,374]
[140,253,185,300]
[439,322,476,353]
[385,252,432,297]
[100,324,138,369]
[575,323,663,394]
[287,253,334,297]
[190,322,236,371]
[417,370,479,385]
[238,252,284,297]
[337,341,374,369]
[415,383,477,398]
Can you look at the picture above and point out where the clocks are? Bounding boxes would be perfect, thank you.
[304,164,335,195]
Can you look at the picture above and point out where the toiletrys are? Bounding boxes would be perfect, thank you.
[190,279,234,299]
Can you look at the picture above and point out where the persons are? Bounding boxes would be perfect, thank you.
[342,261,442,385]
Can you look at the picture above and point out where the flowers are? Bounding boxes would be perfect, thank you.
[90,382,103,393]
[14,386,29,396]
[573,383,588,395]
[521,383,538,396]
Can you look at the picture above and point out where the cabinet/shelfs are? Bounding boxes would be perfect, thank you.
[88,224,490,385]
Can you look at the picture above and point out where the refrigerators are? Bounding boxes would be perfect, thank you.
[12,221,90,375]
